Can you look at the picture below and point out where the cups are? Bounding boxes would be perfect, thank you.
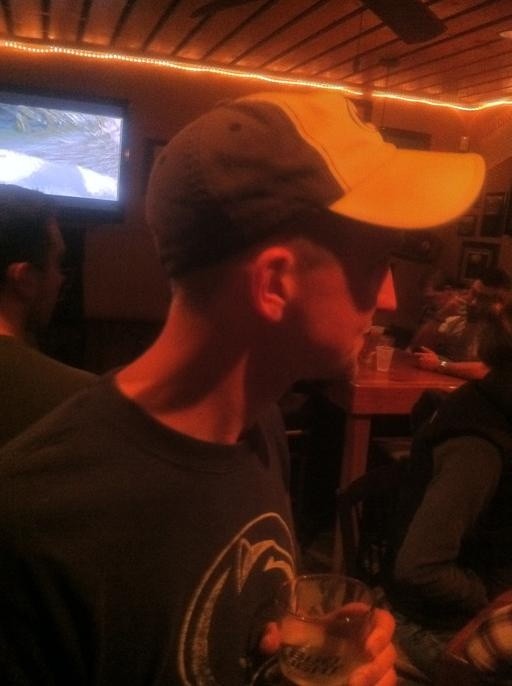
[278,574,375,685]
[375,346,394,374]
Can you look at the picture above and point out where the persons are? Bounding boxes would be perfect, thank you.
[0,91,487,686]
[376,270,512,686]
[0,184,102,448]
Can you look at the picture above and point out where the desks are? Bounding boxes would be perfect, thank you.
[300,345,469,571]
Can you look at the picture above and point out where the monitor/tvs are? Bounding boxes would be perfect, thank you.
[0,82,130,224]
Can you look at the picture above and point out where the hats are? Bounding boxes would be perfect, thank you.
[145,88,486,283]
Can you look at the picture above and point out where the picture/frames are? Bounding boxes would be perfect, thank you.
[455,189,508,285]
